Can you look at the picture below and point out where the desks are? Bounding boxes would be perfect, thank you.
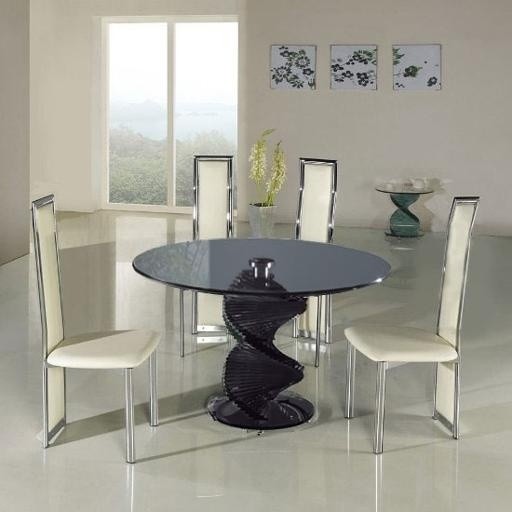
[373,179,440,238]
[132,235,389,430]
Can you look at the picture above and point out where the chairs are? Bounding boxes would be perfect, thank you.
[30,194,163,463]
[180,155,233,357]
[342,194,479,454]
[293,158,337,367]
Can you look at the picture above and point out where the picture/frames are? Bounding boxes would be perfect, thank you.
[391,44,442,90]
[269,43,316,92]
[330,44,378,92]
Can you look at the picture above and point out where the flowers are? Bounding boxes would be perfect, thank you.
[247,128,289,206]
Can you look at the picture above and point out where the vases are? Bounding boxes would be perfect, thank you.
[246,205,280,238]
